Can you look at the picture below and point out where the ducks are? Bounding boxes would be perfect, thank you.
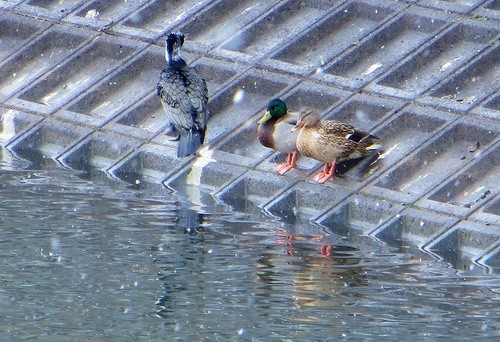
[290,107,383,183]
[156,31,209,158]
[257,99,303,176]
